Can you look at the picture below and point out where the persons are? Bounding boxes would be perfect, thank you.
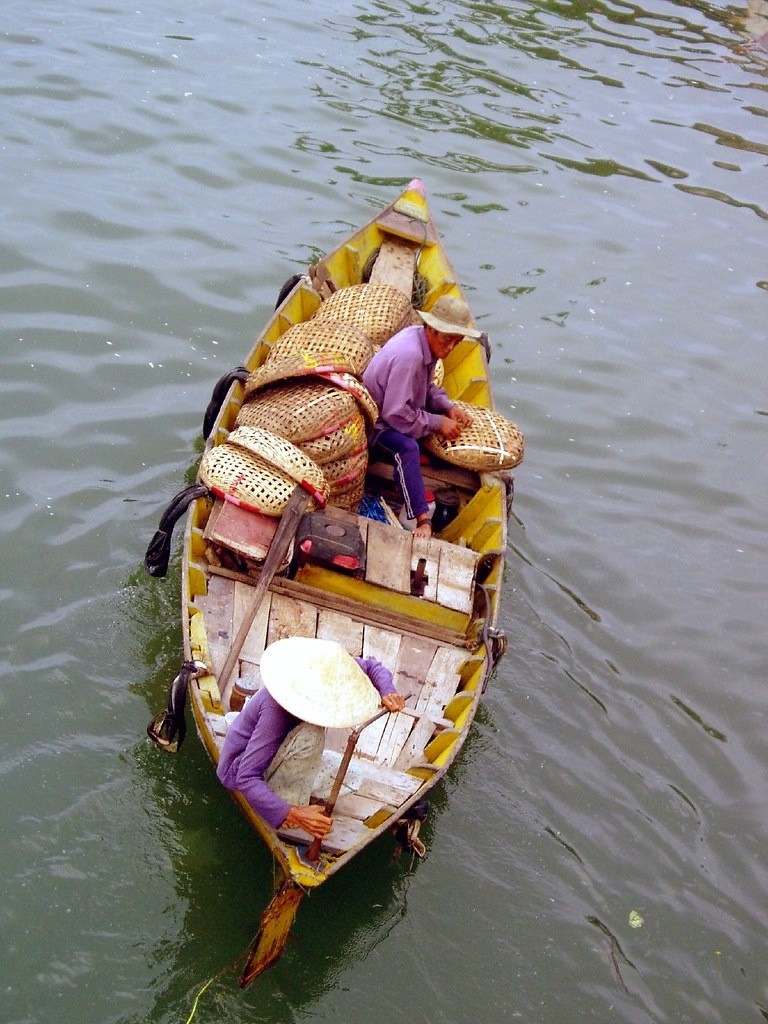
[361,296,482,540]
[215,637,405,840]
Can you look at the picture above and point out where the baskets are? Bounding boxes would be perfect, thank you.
[201,284,414,515]
[422,399,524,471]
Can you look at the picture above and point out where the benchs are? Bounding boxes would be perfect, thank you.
[378,211,437,249]
[370,452,480,492]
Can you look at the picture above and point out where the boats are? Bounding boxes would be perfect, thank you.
[177,174,515,891]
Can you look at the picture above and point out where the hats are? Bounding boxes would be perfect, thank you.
[259,636,380,728]
[416,296,481,339]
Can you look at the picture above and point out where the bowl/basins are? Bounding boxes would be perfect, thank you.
[224,712,240,727]
